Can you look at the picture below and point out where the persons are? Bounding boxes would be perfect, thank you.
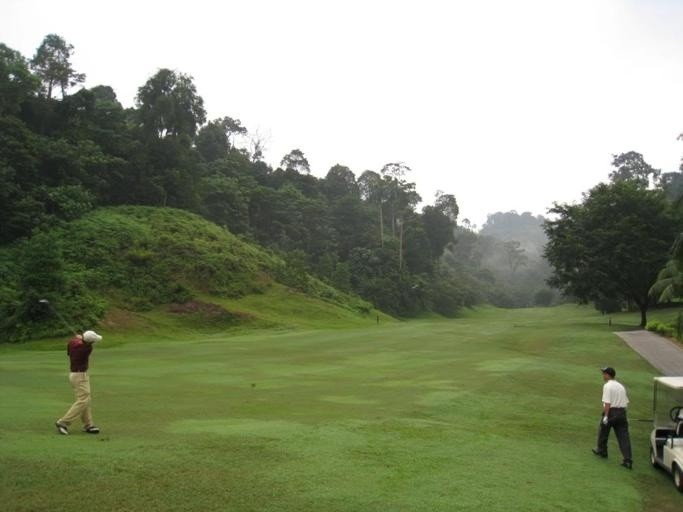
[590,365,633,469]
[53,330,103,436]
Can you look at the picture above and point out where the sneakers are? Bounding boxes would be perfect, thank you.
[54,420,68,435]
[591,448,607,458]
[81,426,99,433]
[620,462,632,469]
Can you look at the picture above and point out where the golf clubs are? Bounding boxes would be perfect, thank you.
[40,299,79,337]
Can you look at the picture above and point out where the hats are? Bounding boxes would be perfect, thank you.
[82,330,102,343]
[600,366,615,376]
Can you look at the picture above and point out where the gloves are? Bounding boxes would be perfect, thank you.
[602,416,608,425]
